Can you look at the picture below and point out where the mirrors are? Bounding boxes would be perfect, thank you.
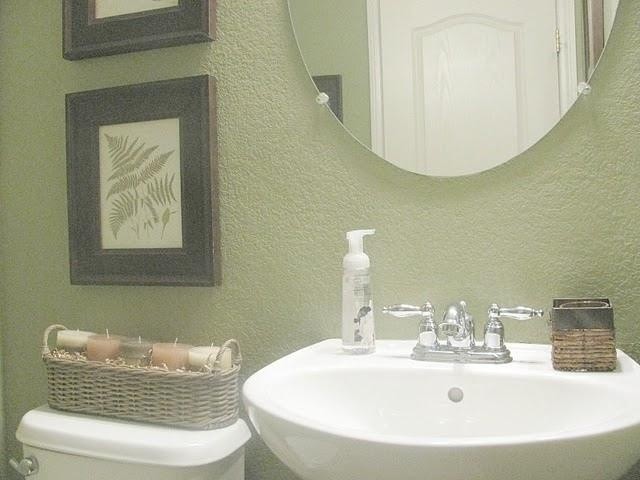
[287,0,620,177]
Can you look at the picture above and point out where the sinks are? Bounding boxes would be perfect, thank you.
[243,338,636,449]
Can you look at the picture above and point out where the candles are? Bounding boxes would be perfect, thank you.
[55,326,231,374]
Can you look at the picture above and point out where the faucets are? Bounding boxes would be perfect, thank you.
[438,300,477,349]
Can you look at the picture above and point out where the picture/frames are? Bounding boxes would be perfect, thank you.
[65,74,222,286]
[62,0,217,59]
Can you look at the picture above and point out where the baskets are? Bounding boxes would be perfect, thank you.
[42,323,241,431]
[549,297,620,373]
